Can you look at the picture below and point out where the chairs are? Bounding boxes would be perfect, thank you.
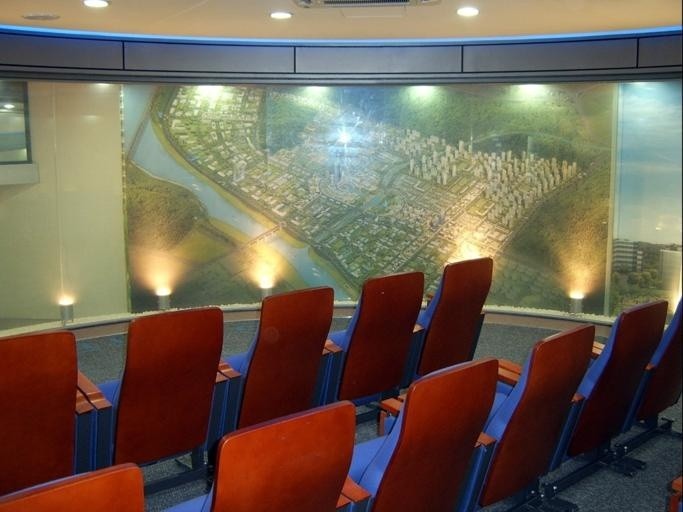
[0,258,683,512]
